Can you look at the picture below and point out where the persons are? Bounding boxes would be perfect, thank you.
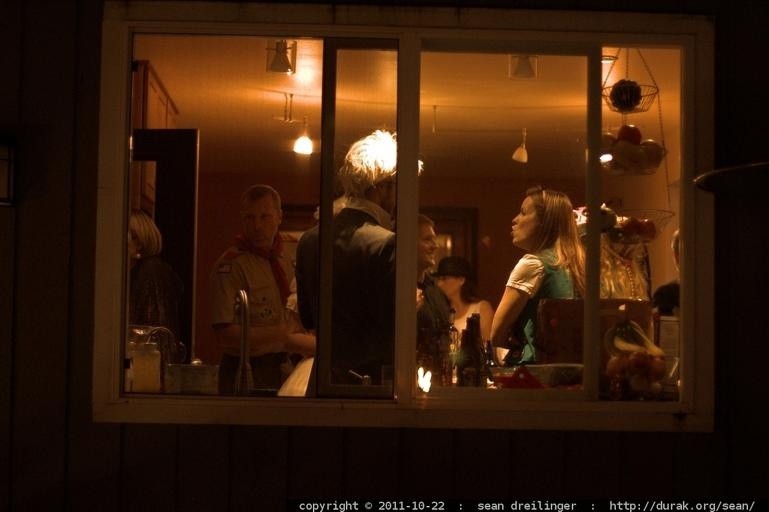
[294,128,425,400]
[488,183,585,365]
[207,182,317,396]
[651,229,681,315]
[430,252,494,354]
[411,214,456,388]
[126,203,182,365]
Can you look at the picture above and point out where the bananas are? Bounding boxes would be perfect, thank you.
[603,320,668,362]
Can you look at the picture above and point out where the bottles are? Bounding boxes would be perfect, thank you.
[131,340,162,393]
[451,312,487,386]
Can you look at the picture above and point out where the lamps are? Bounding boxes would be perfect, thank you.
[512,126,528,165]
[264,37,299,76]
[292,116,313,156]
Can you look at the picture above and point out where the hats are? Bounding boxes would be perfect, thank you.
[427,256,469,277]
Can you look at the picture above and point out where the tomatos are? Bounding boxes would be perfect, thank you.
[617,125,642,145]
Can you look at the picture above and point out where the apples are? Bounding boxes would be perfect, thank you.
[641,138,664,163]
[601,132,615,146]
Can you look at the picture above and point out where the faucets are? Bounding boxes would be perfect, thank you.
[232,289,250,364]
